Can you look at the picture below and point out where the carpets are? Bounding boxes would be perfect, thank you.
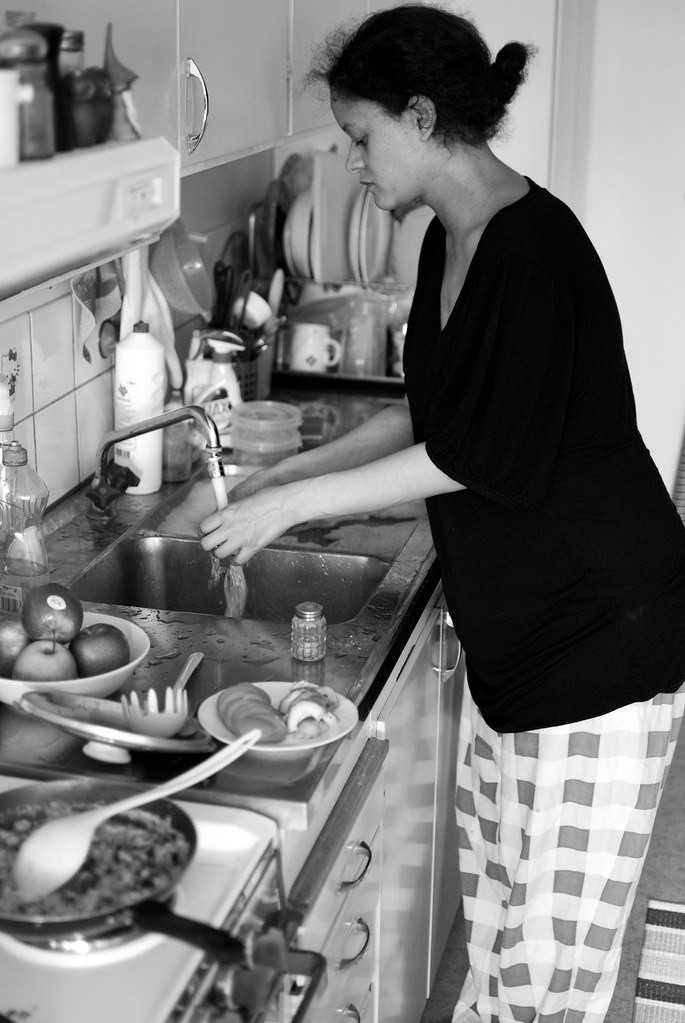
[630,898,685,1022]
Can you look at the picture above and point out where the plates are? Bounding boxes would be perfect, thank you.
[348,184,392,293]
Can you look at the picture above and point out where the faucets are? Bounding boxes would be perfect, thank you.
[85,406,226,521]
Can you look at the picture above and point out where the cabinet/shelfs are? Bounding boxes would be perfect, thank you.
[259,578,468,1023]
[47,1,370,179]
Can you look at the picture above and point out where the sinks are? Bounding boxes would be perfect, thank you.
[66,533,396,626]
[143,461,427,559]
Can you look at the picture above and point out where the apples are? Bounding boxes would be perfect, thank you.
[0,582,130,682]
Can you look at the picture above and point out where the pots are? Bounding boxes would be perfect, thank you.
[0,778,247,966]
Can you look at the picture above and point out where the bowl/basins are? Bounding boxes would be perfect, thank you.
[282,188,314,279]
[149,224,214,323]
[197,681,359,761]
[231,400,303,467]
[0,610,151,708]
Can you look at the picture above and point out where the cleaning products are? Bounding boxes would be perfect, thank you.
[113,321,167,497]
[165,328,246,482]
[0,441,50,615]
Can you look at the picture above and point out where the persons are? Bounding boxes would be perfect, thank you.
[200,4,685,1023]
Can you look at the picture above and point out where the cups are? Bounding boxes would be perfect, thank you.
[288,322,341,373]
[339,293,394,376]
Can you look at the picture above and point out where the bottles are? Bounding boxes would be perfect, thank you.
[0,8,114,167]
[162,391,192,483]
[290,601,328,662]
[0,440,51,621]
[110,320,162,495]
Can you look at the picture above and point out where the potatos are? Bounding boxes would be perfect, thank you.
[217,682,288,745]
